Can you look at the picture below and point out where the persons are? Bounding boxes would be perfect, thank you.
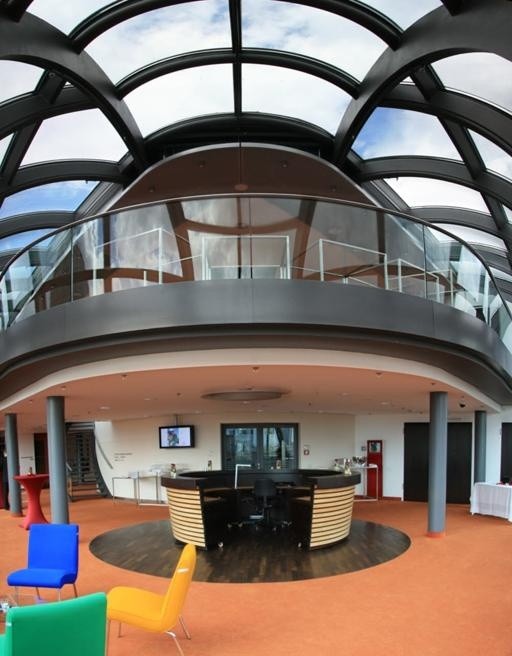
[168,430,179,446]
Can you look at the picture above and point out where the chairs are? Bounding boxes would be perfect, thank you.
[225,477,292,533]
[0,523,197,656]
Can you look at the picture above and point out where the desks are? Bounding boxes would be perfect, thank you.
[469,481,512,522]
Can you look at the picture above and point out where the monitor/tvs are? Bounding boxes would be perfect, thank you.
[159,424,195,448]
